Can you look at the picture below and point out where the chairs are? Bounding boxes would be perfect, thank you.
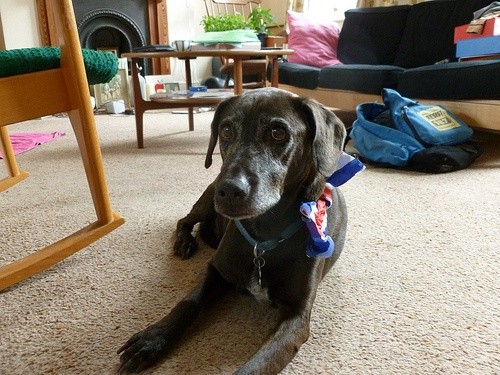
[204,0,269,88]
[0,0,125,292]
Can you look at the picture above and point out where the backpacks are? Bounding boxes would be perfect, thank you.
[349,87,480,173]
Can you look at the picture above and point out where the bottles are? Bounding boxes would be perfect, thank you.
[130,62,147,106]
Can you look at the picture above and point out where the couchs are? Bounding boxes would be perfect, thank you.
[271,0,500,132]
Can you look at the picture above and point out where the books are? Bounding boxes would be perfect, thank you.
[453,16,500,62]
[191,40,262,51]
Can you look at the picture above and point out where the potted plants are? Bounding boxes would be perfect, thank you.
[248,7,280,47]
[199,12,261,50]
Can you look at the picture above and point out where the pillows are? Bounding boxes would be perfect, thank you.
[286,9,342,67]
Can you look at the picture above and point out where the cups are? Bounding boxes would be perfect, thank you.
[106,99,125,113]
[266,36,284,47]
[188,86,207,98]
[171,40,192,52]
[165,83,180,94]
[241,42,261,51]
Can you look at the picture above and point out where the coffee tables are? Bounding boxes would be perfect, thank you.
[119,50,295,150]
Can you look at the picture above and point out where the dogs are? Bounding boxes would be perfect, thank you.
[113,85,349,375]
